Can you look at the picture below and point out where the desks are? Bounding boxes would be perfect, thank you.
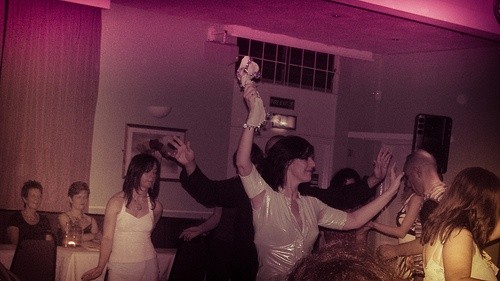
[56,246,176,281]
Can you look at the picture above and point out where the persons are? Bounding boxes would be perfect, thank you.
[420,167,500,281]
[236,56,266,129]
[364,150,448,281]
[166,135,392,281]
[82,153,164,281]
[236,83,405,281]
[57,182,98,246]
[5,181,53,246]
[287,237,401,281]
[317,167,369,250]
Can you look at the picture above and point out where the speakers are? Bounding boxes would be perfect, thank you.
[413,114,453,173]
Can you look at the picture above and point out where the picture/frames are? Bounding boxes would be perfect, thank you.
[123,124,188,182]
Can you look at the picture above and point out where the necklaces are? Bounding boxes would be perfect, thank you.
[136,204,142,210]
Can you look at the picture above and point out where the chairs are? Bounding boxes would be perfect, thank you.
[0,240,56,281]
[167,236,208,281]
